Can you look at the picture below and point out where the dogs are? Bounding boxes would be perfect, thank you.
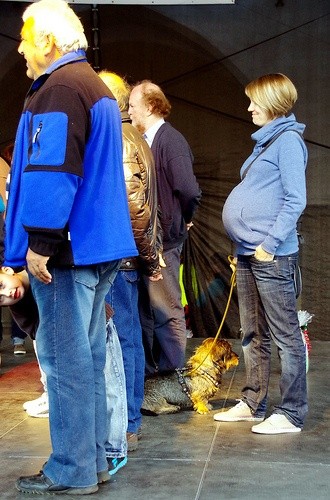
[139,336,242,417]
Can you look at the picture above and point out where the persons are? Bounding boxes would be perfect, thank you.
[5,0,141,496]
[7,316,28,355]
[97,71,165,455]
[22,340,49,418]
[0,266,130,476]
[213,73,310,436]
[127,81,201,375]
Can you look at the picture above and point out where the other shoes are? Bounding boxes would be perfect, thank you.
[15,469,99,494]
[14,344,27,354]
[22,392,49,417]
[106,456,128,476]
[40,469,112,483]
[127,423,143,452]
[252,414,302,434]
[213,399,265,422]
